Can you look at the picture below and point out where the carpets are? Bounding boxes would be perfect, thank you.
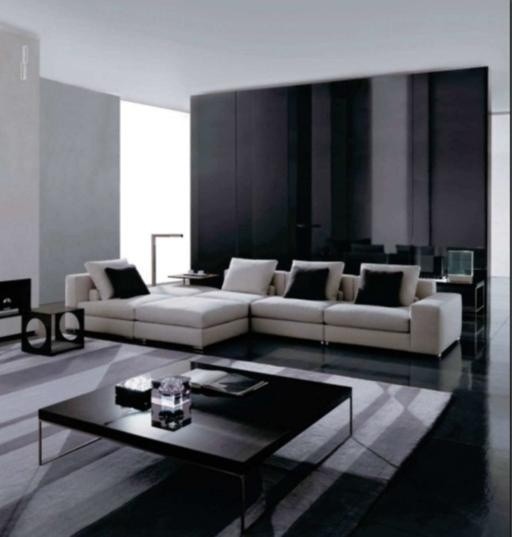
[0,335,454,537]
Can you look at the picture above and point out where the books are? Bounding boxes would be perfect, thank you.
[182,366,268,397]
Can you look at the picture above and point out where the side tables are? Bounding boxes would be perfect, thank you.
[21,303,84,357]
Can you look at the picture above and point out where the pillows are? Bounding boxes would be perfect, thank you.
[356,262,420,308]
[220,258,278,296]
[353,269,405,307]
[283,265,332,302]
[84,258,132,301]
[104,264,152,300]
[282,259,346,303]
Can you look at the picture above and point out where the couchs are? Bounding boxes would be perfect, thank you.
[64,270,463,362]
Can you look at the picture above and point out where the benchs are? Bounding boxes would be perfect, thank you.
[421,271,487,314]
